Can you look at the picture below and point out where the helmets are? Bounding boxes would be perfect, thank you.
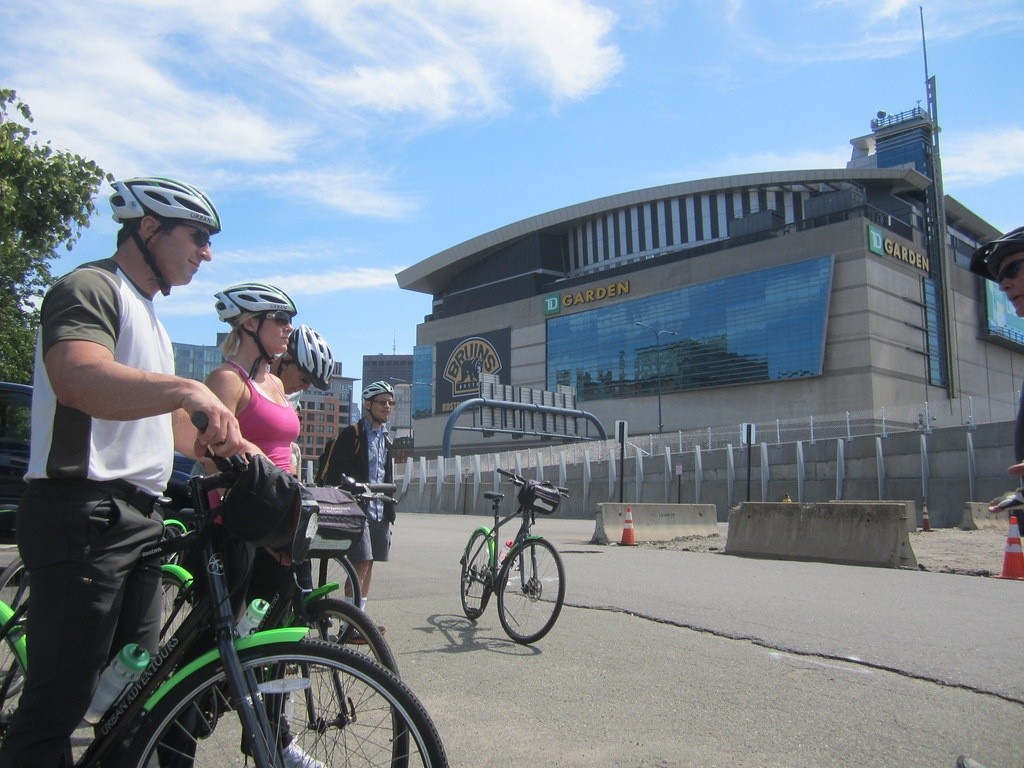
[361,380,394,400]
[287,322,334,391]
[212,281,298,323]
[972,226,1024,283]
[107,174,223,236]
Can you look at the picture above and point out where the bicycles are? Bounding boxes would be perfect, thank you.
[1,407,455,768]
[459,467,571,646]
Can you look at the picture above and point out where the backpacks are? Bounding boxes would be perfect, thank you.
[316,423,359,487]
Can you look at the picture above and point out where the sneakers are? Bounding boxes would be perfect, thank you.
[337,624,387,644]
[272,739,327,768]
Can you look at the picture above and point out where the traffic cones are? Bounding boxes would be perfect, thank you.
[993,515,1024,581]
[616,506,639,547]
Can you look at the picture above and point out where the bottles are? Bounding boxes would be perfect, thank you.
[83,644,150,724]
[234,599,270,639]
[496,541,512,567]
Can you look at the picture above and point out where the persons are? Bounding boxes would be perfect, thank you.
[270,325,335,628]
[206,281,326,768]
[0,176,274,768]
[968,227,1024,536]
[320,381,395,645]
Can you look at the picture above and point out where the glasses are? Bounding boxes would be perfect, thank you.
[370,400,396,407]
[293,357,310,386]
[157,218,213,248]
[270,311,294,326]
[995,258,1024,284]
